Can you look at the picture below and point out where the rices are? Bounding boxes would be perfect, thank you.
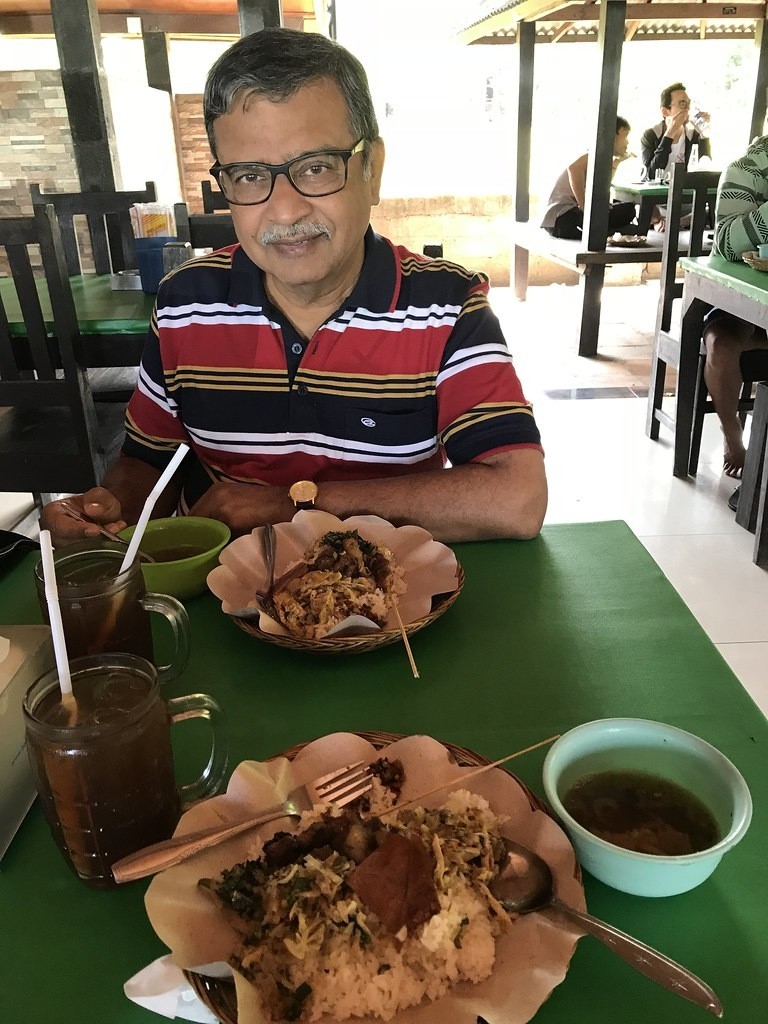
[279,538,407,640]
[233,774,499,1024]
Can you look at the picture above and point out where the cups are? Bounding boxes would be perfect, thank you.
[757,244,768,260]
[23,652,230,890]
[35,539,193,684]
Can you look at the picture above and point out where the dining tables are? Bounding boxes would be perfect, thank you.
[1,521,767,1024]
[610,175,732,243]
[0,271,170,408]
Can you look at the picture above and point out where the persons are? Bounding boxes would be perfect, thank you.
[706,134,768,481]
[541,116,639,242]
[640,83,713,232]
[38,28,547,549]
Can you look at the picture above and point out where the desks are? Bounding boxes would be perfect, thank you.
[670,243,767,537]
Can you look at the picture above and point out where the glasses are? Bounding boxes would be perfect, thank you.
[669,100,691,109]
[209,139,367,206]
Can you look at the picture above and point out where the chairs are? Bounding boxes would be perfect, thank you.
[2,205,138,491]
[26,176,237,403]
[652,166,749,479]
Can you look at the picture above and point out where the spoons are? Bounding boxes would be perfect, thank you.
[261,521,276,610]
[488,838,724,1019]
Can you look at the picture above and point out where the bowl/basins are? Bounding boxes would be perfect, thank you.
[105,516,231,600]
[545,718,754,897]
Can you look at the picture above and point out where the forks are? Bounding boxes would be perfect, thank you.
[110,760,374,884]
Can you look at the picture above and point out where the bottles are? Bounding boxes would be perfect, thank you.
[688,108,714,139]
[687,143,699,173]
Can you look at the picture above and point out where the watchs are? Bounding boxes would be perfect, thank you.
[287,478,318,511]
